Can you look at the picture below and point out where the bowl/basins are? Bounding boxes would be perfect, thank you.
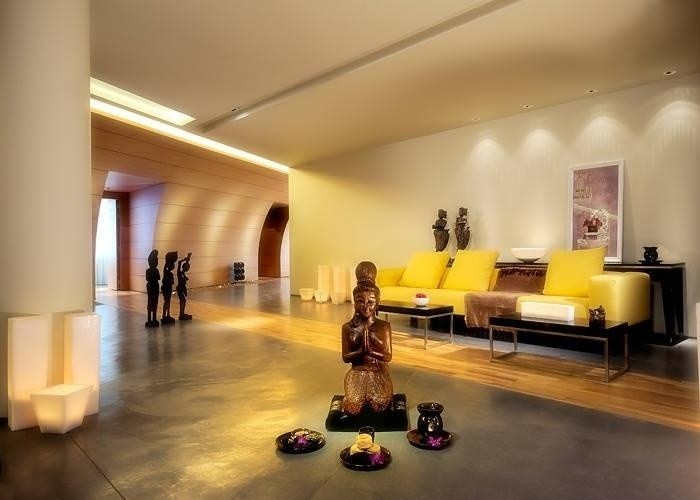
[412,297,430,307]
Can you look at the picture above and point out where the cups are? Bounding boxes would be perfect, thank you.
[359,426,374,444]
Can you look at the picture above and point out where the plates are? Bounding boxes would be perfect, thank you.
[515,257,541,263]
[276,430,325,454]
[407,429,451,451]
[340,446,391,471]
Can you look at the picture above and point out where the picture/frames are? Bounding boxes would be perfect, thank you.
[567,157,627,266]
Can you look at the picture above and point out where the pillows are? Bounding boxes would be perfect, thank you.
[397,249,452,289]
[541,244,609,298]
[442,249,500,292]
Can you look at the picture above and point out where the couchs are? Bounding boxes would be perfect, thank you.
[375,266,653,359]
